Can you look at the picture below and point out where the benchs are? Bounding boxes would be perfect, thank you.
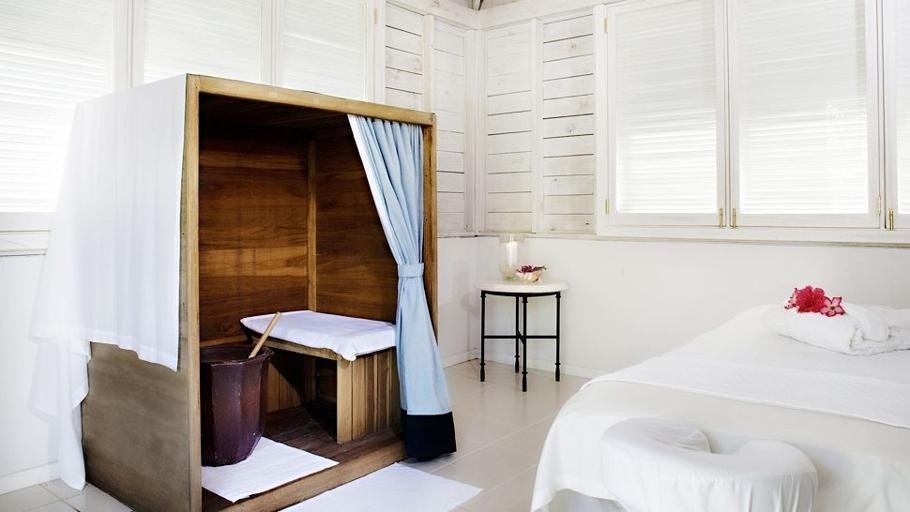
[239,309,401,447]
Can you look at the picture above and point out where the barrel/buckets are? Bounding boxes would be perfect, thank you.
[200,343,274,466]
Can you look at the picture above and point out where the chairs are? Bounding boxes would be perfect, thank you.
[598,417,818,512]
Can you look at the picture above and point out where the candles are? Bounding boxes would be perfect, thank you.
[506,235,516,270]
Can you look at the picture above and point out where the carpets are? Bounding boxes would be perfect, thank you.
[275,462,483,511]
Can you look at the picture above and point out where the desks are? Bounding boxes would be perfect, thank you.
[475,279,567,392]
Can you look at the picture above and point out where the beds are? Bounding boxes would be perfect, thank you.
[529,303,910,512]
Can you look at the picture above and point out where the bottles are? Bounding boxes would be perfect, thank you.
[495,232,522,281]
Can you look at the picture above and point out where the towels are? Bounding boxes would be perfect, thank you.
[767,298,910,356]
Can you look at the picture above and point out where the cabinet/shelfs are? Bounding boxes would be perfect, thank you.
[62,74,439,512]
